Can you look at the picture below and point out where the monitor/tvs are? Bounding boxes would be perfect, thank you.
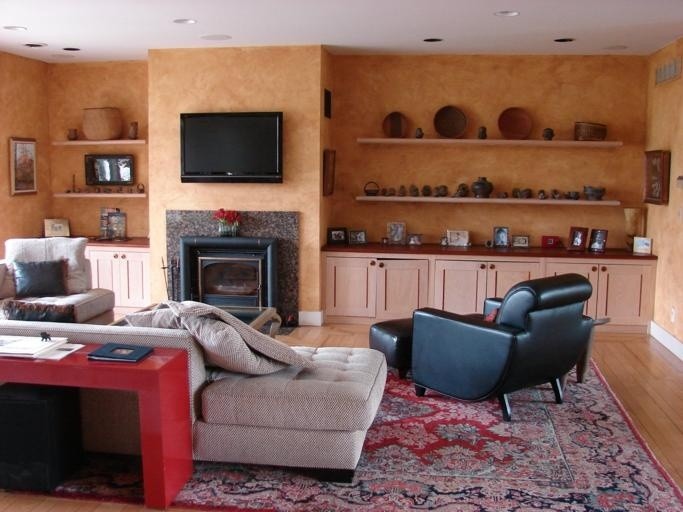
[179,111,283,184]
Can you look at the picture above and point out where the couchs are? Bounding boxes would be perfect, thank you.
[0,298,388,484]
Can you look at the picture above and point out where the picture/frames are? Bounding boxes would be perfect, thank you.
[493,226,509,248]
[511,235,530,249]
[349,230,367,247]
[566,226,589,251]
[386,221,407,245]
[8,137,37,196]
[445,229,471,247]
[327,226,347,245]
[588,228,609,253]
[43,218,70,237]
[633,237,652,254]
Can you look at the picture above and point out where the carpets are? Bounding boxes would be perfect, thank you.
[0,357,683,512]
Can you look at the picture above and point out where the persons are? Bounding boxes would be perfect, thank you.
[495,229,506,245]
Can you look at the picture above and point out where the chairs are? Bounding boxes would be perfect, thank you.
[0,259,115,325]
[411,273,594,422]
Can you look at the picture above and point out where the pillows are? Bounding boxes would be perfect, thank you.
[124,308,289,376]
[4,237,89,295]
[11,259,67,299]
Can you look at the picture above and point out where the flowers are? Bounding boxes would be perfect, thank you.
[211,208,244,235]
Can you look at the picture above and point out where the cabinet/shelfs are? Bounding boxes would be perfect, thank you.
[85,247,149,308]
[542,258,655,333]
[430,254,546,316]
[355,138,623,207]
[321,252,436,323]
[51,139,148,199]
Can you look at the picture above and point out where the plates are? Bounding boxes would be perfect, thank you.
[434,105,467,137]
[382,112,408,137]
[498,107,533,140]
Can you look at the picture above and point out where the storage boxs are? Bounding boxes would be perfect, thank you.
[81,107,123,141]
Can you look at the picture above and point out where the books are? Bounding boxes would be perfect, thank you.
[87,342,154,362]
[0,336,85,361]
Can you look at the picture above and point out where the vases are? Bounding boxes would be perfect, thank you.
[623,208,642,252]
[219,221,237,236]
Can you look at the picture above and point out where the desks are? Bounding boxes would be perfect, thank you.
[0,336,194,510]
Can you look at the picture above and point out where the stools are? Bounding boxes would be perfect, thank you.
[369,314,484,380]
